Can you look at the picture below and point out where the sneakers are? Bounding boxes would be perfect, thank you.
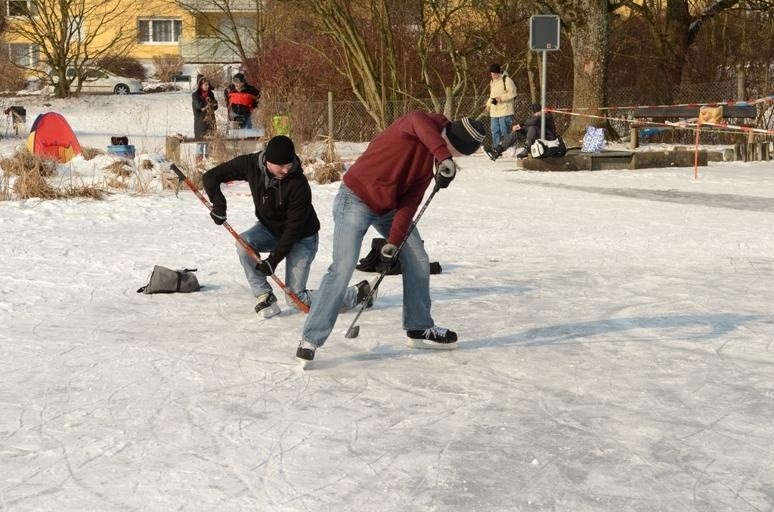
[517,147,530,158]
[407,325,457,343]
[296,339,316,360]
[357,280,372,308]
[255,292,277,312]
[484,143,499,159]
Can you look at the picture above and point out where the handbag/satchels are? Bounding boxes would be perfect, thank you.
[581,127,606,153]
[530,136,566,158]
[143,264,200,294]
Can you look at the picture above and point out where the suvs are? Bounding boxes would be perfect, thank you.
[46,65,144,96]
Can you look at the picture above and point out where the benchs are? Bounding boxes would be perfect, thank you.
[164,133,269,164]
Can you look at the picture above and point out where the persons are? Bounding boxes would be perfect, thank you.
[482,103,557,163]
[483,63,518,158]
[200,134,373,315]
[295,108,490,363]
[190,74,217,140]
[220,72,259,130]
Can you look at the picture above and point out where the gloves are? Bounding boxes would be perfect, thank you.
[436,158,455,188]
[491,98,497,105]
[381,244,397,266]
[256,258,275,276]
[209,206,227,225]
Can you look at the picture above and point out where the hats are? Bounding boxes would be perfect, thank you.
[532,103,541,112]
[232,73,244,82]
[264,135,294,165]
[490,63,500,73]
[446,117,485,155]
